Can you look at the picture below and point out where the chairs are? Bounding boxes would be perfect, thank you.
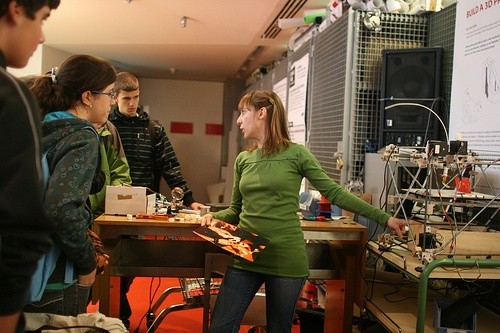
[202,250,267,333]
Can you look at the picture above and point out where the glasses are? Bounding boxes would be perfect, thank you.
[93,90,115,99]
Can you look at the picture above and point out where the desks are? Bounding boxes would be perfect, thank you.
[94,212,370,333]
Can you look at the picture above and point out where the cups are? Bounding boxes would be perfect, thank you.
[418,232,436,249]
[200,206,210,217]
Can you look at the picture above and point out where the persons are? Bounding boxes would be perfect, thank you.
[20,55,116,318]
[86,121,132,217]
[108,72,205,328]
[0,0,60,333]
[200,89,407,333]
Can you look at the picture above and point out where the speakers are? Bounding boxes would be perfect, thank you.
[379,47,443,134]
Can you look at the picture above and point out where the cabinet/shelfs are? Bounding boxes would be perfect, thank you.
[366,238,500,333]
[364,153,475,241]
